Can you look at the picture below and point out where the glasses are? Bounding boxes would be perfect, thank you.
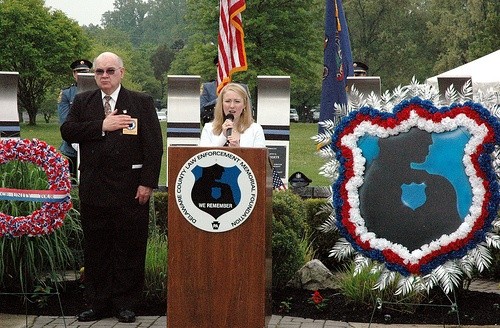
[95,67,121,75]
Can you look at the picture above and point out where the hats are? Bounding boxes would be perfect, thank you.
[288,172,312,188]
[353,61,368,76]
[70,59,93,71]
[213,57,218,65]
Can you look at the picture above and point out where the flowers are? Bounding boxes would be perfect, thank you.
[0,136,73,238]
[310,74,500,301]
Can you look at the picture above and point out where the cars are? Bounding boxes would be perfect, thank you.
[303,104,322,122]
[290,109,300,123]
[155,107,169,121]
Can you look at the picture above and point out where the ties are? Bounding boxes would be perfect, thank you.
[104,95,112,119]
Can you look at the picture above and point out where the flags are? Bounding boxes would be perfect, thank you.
[316,0,354,151]
[271,168,287,192]
[216,0,249,96]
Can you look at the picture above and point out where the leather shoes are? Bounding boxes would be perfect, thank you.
[79,309,113,321]
[116,307,136,322]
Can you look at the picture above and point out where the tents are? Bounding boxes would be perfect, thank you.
[425,50,500,106]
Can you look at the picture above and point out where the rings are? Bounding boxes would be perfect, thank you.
[226,123,229,126]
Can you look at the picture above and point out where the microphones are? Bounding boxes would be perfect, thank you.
[226,113,234,135]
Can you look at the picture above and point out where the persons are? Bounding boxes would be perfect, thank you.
[60,53,164,325]
[200,54,248,124]
[352,61,369,76]
[57,59,92,185]
[199,83,265,148]
